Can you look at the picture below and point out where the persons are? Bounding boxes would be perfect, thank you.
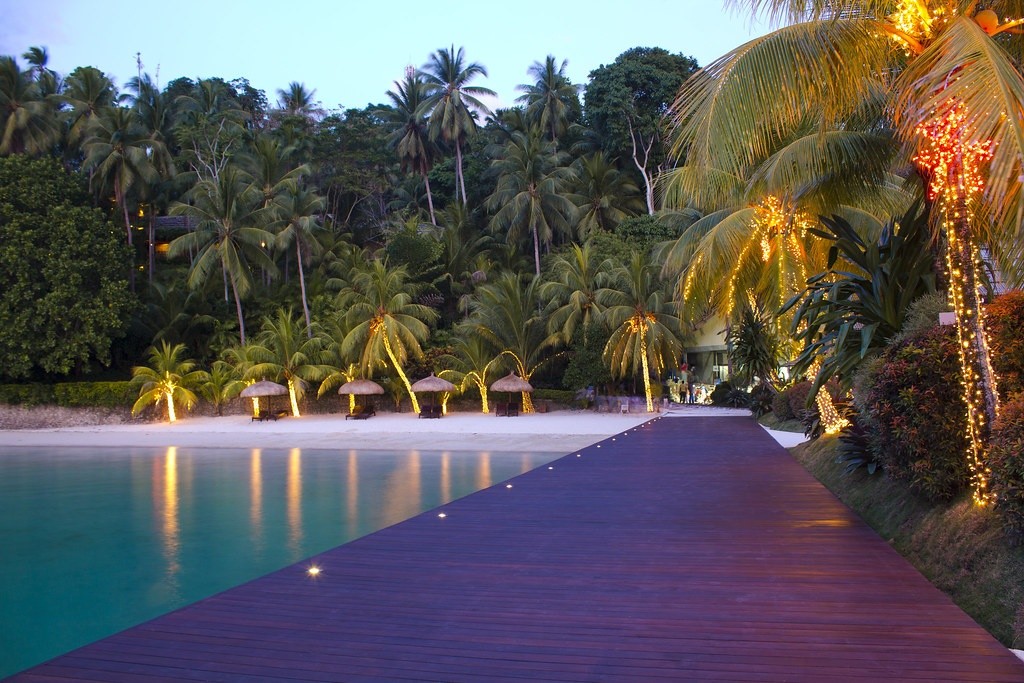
[679,378,710,404]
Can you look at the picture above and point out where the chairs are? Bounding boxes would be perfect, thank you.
[430,405,444,418]
[507,402,519,417]
[266,410,288,422]
[496,403,508,417]
[419,404,431,419]
[252,411,269,423]
[346,406,364,420]
[355,406,376,420]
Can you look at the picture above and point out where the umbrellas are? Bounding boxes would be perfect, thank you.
[240,380,288,416]
[490,371,533,402]
[410,373,454,404]
[338,380,385,406]
